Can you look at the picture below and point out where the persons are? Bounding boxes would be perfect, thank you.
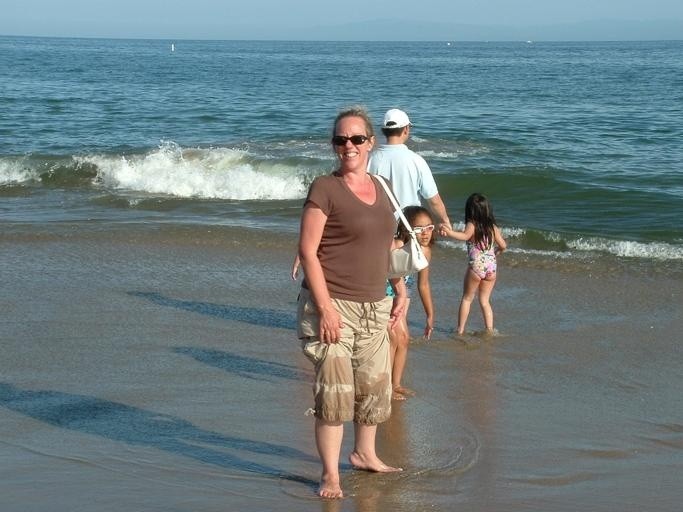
[438,192,507,335]
[366,107,452,338]
[384,205,434,401]
[292,250,300,282]
[296,104,408,500]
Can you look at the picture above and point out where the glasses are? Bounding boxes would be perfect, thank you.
[414,224,435,234]
[332,135,370,146]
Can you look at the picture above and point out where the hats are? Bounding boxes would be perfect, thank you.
[381,109,414,129]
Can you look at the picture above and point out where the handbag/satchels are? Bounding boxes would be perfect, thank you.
[387,235,429,281]
